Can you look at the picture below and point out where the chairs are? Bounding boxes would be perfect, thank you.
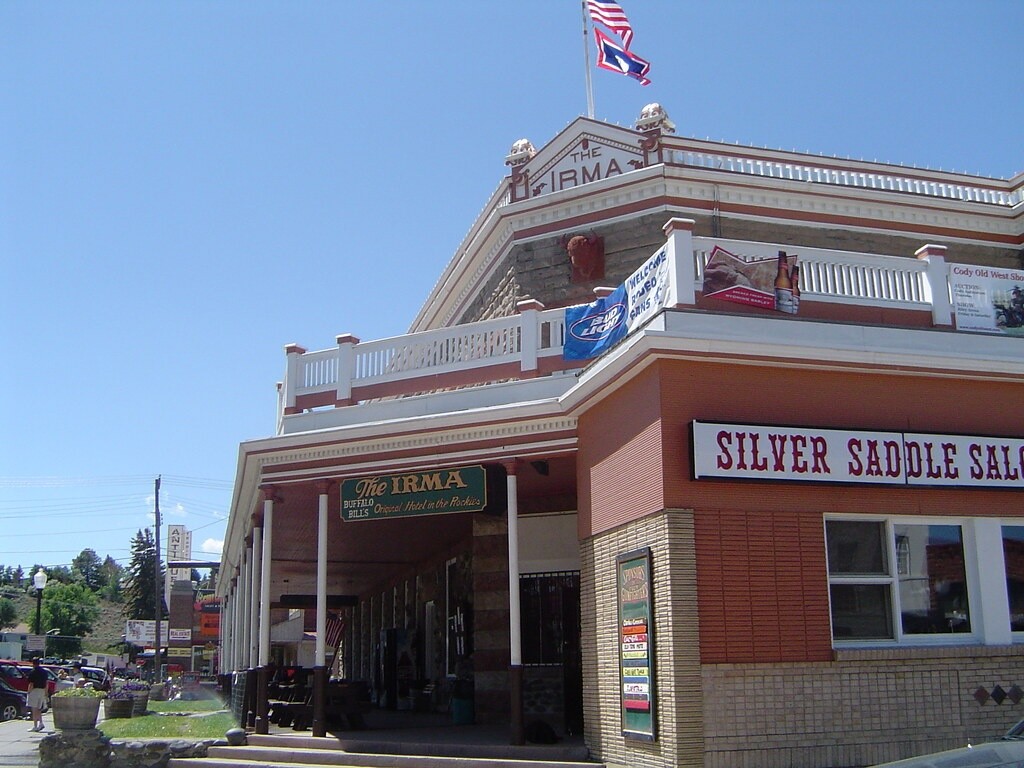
[266,681,357,731]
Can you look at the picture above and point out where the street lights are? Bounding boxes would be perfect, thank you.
[32,568,47,637]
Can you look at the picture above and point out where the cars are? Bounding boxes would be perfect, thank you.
[0,659,139,725]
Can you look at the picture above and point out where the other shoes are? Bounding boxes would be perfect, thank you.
[35,723,45,732]
[27,727,39,732]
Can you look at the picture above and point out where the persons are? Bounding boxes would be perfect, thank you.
[72,663,83,688]
[521,639,582,736]
[26,657,48,732]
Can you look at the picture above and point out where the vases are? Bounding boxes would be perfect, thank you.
[51,683,108,730]
[130,691,150,714]
[104,699,135,720]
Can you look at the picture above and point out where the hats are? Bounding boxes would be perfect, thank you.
[72,663,82,670]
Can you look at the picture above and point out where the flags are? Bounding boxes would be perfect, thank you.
[326,611,344,648]
[586,0,652,87]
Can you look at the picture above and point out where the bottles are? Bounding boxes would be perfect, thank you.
[790,265,801,315]
[773,250,793,314]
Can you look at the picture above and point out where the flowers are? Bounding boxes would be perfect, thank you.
[105,690,133,699]
[121,683,151,691]
